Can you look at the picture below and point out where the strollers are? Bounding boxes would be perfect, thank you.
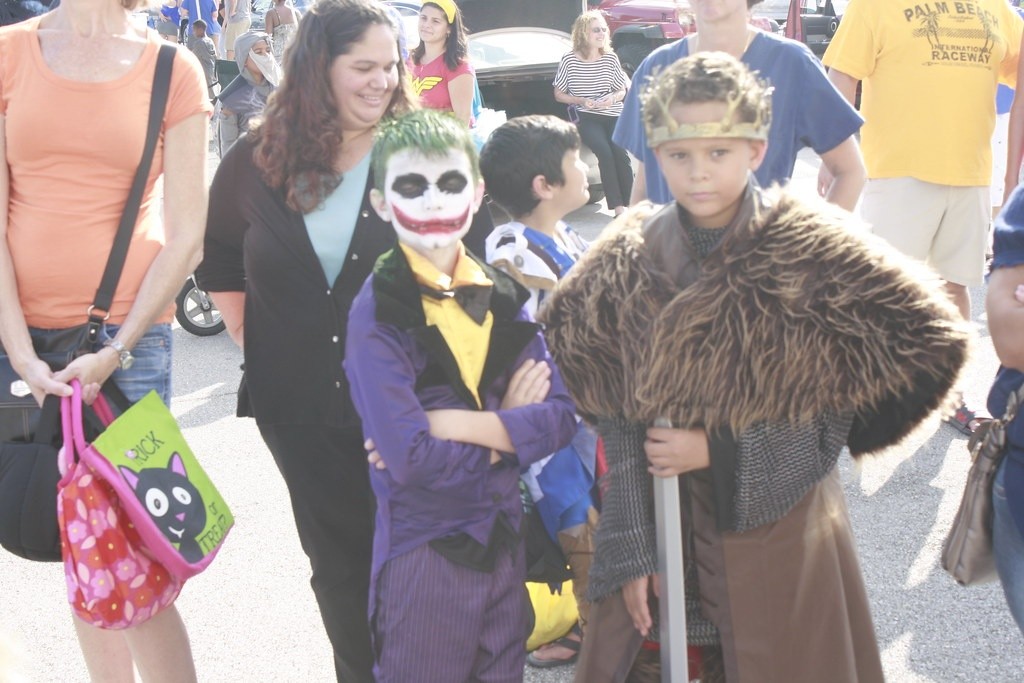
[176,274,227,338]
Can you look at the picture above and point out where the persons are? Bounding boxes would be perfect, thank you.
[817,0,1024,435]
[402,0,474,132]
[539,50,968,683]
[194,0,407,683]
[0,0,303,159]
[985,182,1024,632]
[553,13,633,217]
[0,0,212,683]
[347,107,580,683]
[477,113,612,593]
[612,0,868,214]
[994,31,1024,219]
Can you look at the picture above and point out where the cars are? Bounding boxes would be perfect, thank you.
[250,0,841,205]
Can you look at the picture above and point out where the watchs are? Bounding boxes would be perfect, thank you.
[102,338,133,369]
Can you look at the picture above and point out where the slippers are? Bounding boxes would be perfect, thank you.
[946,399,989,437]
[528,623,583,667]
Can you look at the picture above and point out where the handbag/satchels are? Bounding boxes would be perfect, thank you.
[940,416,1010,588]
[1,355,236,630]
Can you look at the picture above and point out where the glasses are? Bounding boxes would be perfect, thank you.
[586,27,608,34]
